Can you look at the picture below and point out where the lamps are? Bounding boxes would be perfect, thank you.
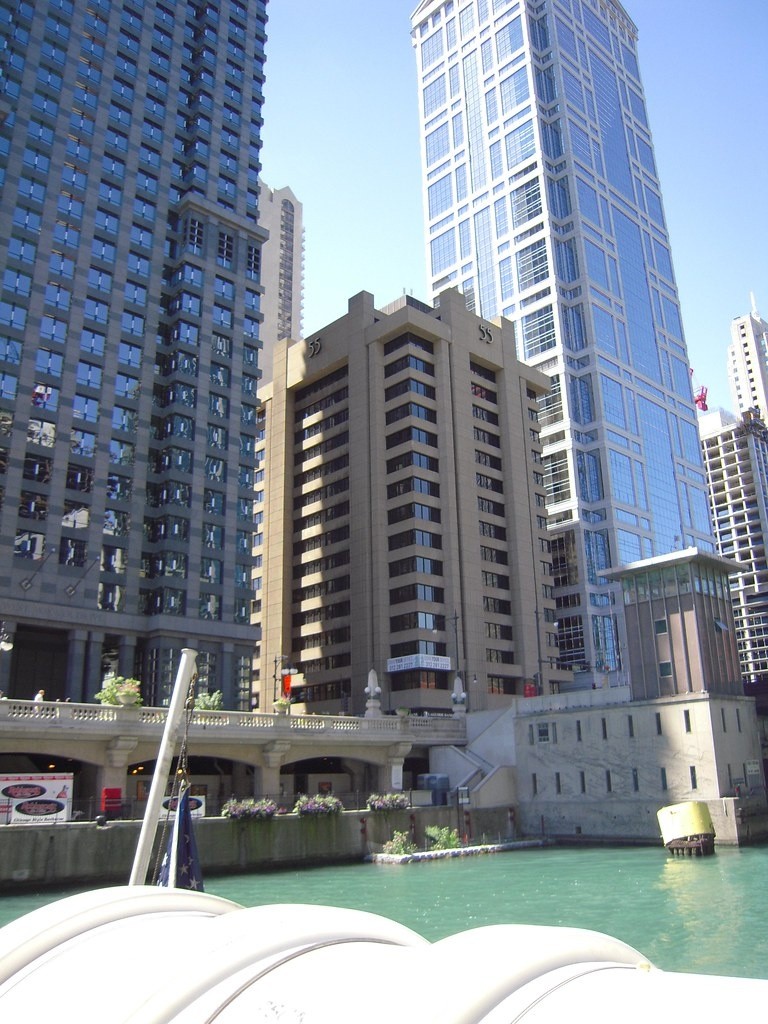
[451,692,458,704]
[460,693,466,704]
[374,687,381,699]
[364,687,371,699]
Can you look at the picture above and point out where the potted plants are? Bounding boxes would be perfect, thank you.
[117,684,139,704]
[395,704,410,715]
[271,696,291,713]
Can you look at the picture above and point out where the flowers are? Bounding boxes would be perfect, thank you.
[365,793,410,812]
[220,797,277,815]
[292,793,345,817]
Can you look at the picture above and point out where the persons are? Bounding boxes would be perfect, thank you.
[735,784,741,797]
[35,690,44,711]
[56,698,71,702]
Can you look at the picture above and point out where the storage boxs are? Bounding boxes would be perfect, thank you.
[418,773,450,806]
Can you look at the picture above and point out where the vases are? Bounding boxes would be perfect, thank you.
[370,804,375,812]
[231,811,274,820]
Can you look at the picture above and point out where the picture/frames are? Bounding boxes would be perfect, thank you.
[136,780,208,799]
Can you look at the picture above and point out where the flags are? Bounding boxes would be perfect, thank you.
[156,779,204,892]
